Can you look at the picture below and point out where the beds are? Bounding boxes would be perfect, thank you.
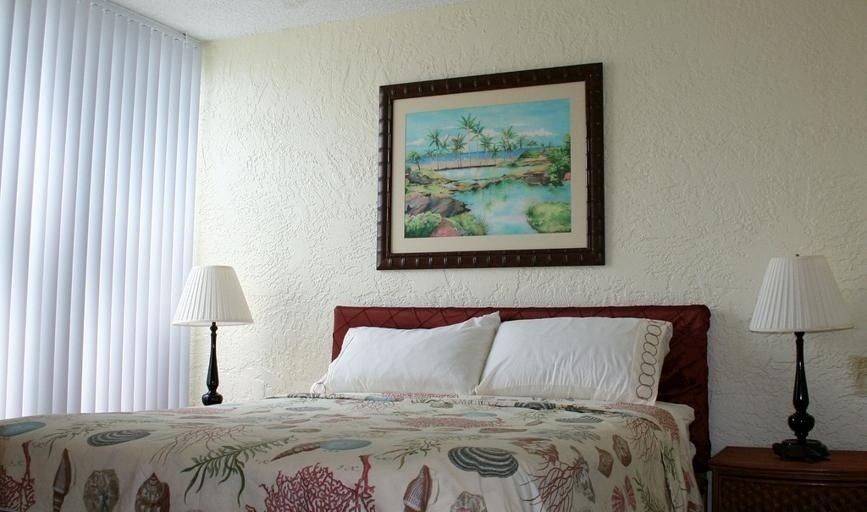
[0,303,712,512]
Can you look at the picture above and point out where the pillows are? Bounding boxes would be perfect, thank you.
[308,311,501,395]
[474,316,673,406]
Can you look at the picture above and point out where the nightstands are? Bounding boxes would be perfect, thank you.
[707,445,866,511]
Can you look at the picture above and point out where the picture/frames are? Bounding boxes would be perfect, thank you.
[375,62,608,271]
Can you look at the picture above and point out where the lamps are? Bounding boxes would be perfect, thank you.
[747,254,854,461]
[171,264,255,408]
